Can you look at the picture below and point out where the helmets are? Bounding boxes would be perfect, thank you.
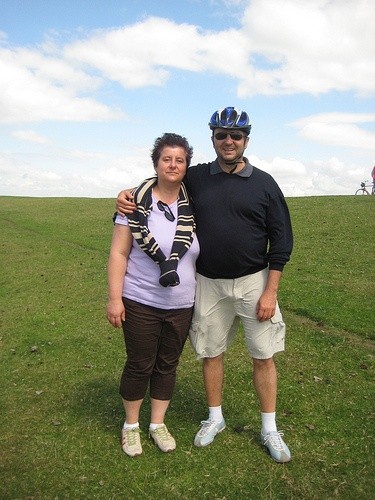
[208,107,252,135]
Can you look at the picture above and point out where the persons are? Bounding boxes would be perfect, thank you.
[108,132,201,457]
[115,107,292,464]
[370,165,375,195]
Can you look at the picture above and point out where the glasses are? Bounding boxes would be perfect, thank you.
[213,132,246,141]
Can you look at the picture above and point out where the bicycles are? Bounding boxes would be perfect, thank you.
[354,179,375,196]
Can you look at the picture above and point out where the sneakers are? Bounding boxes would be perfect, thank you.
[193,416,226,446]
[148,423,176,453]
[121,423,144,458]
[261,426,291,462]
